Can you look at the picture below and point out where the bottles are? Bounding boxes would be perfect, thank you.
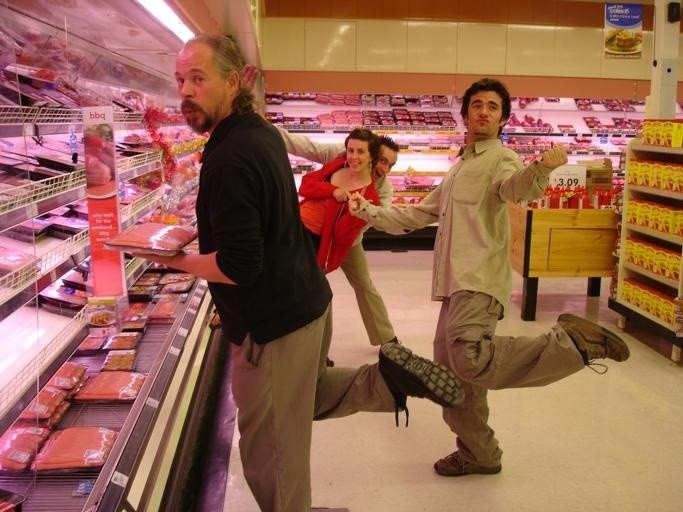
[593,183,620,209]
[523,183,586,209]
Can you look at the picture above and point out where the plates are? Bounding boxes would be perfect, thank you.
[605,42,641,55]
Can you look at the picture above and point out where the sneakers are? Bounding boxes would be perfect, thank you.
[378,342,466,428]
[432,450,500,476]
[557,312,629,375]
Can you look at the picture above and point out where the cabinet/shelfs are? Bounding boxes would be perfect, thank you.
[609,139,683,363]
[264,90,646,251]
[505,200,621,320]
[0,2,223,512]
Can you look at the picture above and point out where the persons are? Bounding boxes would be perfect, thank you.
[273,123,402,366]
[348,78,630,476]
[299,127,382,273]
[130,30,467,512]
